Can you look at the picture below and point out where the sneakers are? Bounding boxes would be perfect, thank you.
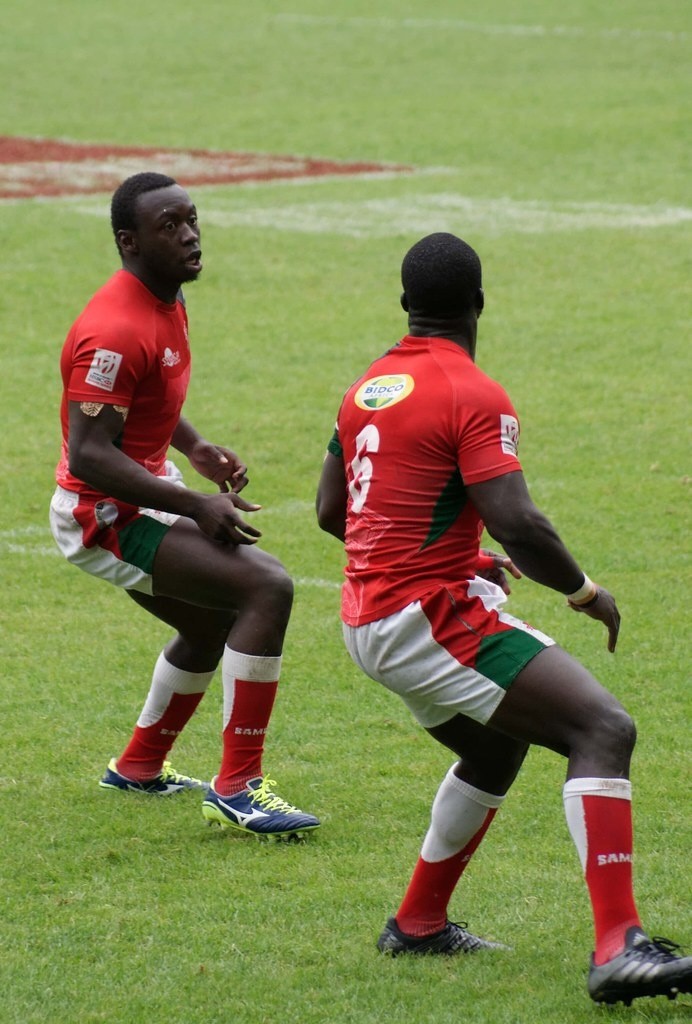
[99,757,210,797]
[587,925,692,1006]
[376,917,513,959]
[202,776,321,841]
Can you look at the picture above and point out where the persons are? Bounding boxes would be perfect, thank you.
[49,171,320,843]
[316,233,692,1006]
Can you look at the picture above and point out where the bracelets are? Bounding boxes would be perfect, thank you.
[566,572,600,608]
